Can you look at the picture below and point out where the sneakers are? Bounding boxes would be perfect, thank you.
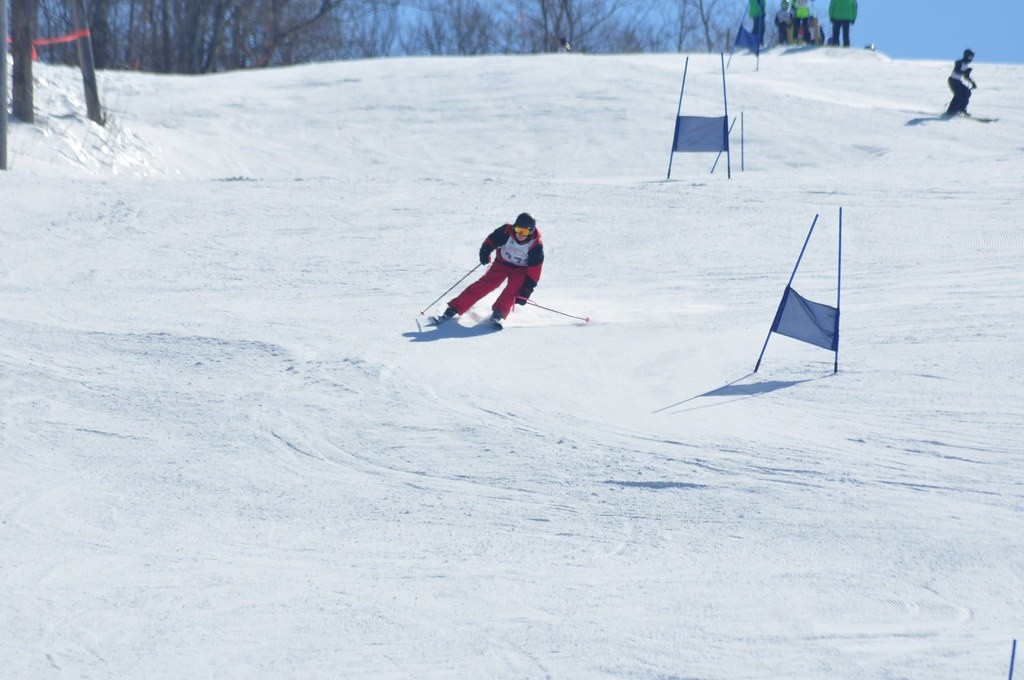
[489,310,504,325]
[441,307,456,319]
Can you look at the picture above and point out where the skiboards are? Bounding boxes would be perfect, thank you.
[430,310,502,330]
[940,112,1001,124]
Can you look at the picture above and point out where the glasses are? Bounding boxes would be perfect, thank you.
[514,226,530,236]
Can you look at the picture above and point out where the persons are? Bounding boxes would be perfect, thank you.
[947,50,977,116]
[749,0,766,47]
[557,39,571,53]
[774,0,825,47]
[829,0,857,48]
[443,213,544,324]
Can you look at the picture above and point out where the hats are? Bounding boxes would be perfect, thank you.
[512,212,536,235]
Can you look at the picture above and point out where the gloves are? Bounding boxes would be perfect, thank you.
[515,285,533,306]
[479,249,491,265]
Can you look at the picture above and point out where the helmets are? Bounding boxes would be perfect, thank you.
[963,50,975,62]
